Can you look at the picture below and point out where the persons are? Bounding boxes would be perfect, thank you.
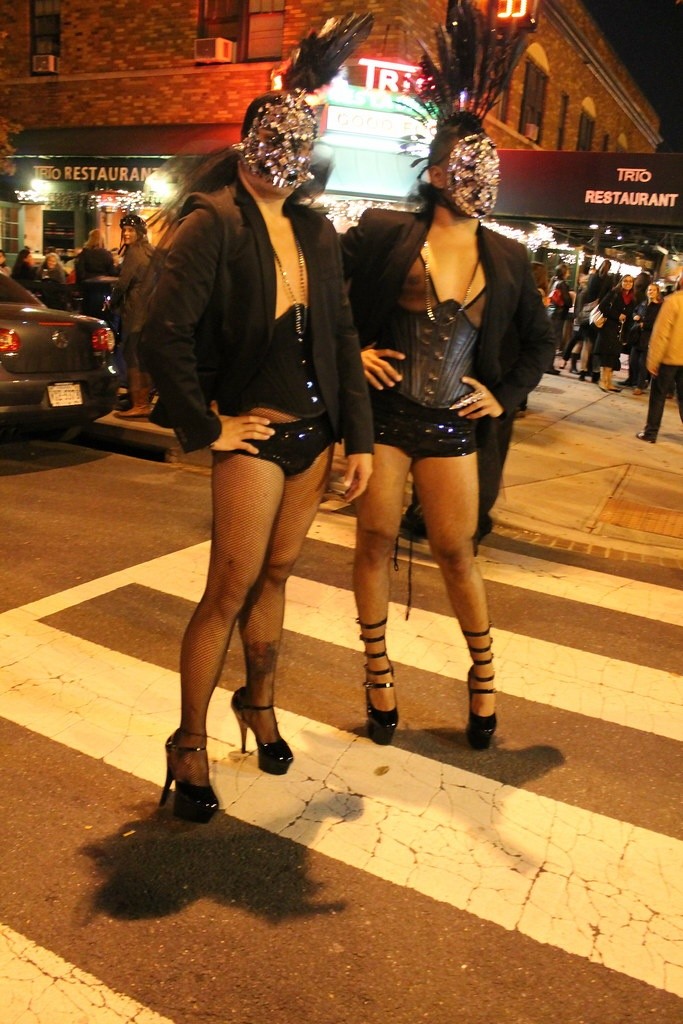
[105,214,165,418]
[73,228,115,319]
[63,259,76,284]
[0,250,12,276]
[132,88,376,826]
[11,249,42,294]
[340,110,557,748]
[636,274,683,444]
[531,245,666,395]
[34,251,66,309]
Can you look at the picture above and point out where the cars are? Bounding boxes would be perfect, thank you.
[0,273,118,444]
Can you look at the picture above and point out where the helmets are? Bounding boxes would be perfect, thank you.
[119,215,147,233]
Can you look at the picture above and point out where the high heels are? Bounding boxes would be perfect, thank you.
[231,687,293,776]
[160,728,219,824]
[466,664,498,749]
[364,665,399,745]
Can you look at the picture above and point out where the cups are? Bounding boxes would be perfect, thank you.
[42,269,49,279]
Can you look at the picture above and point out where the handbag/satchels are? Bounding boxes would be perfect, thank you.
[627,301,644,345]
[589,302,608,329]
[549,281,565,307]
[578,298,599,325]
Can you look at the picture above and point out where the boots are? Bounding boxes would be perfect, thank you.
[115,366,154,417]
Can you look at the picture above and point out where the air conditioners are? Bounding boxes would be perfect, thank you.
[524,123,540,140]
[31,54,56,74]
[192,37,238,65]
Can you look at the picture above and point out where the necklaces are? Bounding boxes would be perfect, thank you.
[273,235,308,334]
[423,241,480,327]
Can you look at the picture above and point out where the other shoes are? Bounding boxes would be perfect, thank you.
[545,367,560,374]
[579,373,585,380]
[665,392,673,398]
[633,387,642,396]
[592,373,600,382]
[559,359,566,369]
[607,384,622,392]
[599,383,608,393]
[618,380,632,386]
[637,431,656,444]
[569,369,579,374]
